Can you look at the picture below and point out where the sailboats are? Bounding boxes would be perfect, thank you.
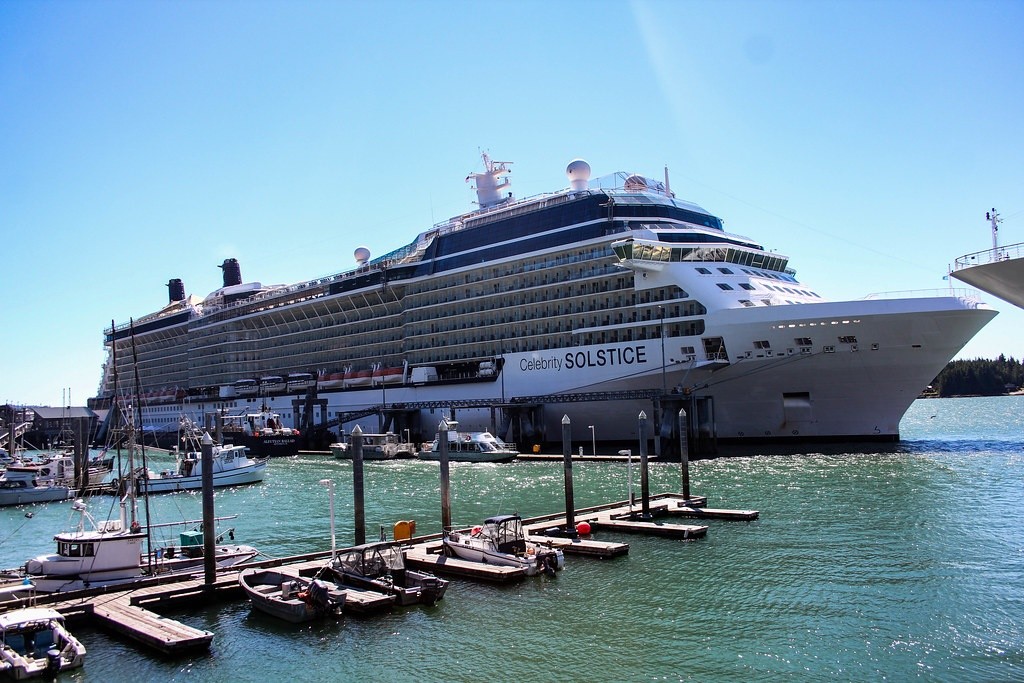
[238,567,347,624]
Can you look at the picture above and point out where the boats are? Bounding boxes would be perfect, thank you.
[0,318,270,603]
[260,376,286,393]
[329,431,418,460]
[443,498,565,578]
[0,608,87,682]
[317,372,344,387]
[321,539,449,607]
[344,370,372,384]
[234,379,259,395]
[286,374,316,391]
[178,406,300,457]
[372,366,405,383]
[417,409,520,463]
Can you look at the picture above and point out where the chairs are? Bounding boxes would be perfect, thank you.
[5,627,54,658]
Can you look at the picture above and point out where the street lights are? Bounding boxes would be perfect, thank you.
[404,429,410,443]
[589,425,595,456]
[618,450,632,511]
[317,478,335,560]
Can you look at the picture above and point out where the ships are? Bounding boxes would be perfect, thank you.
[950,205,1024,311]
[86,147,999,457]
